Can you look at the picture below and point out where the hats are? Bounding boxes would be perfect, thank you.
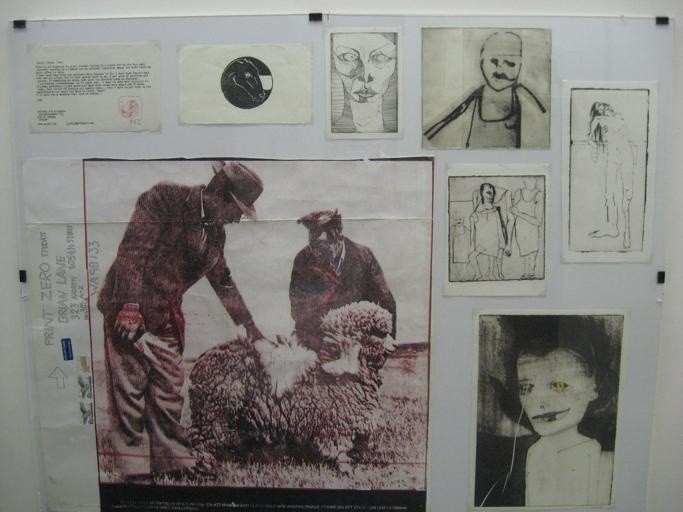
[209,159,262,222]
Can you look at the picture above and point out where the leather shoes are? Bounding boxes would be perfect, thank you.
[130,475,158,486]
[170,461,220,484]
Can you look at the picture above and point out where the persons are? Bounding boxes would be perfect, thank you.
[287,207,395,369]
[472,176,515,280]
[477,314,612,506]
[513,175,543,280]
[331,32,398,133]
[422,30,546,149]
[468,183,507,281]
[92,159,277,487]
[448,212,472,265]
[584,99,634,250]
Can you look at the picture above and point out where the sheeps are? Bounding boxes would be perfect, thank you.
[189,300,399,479]
